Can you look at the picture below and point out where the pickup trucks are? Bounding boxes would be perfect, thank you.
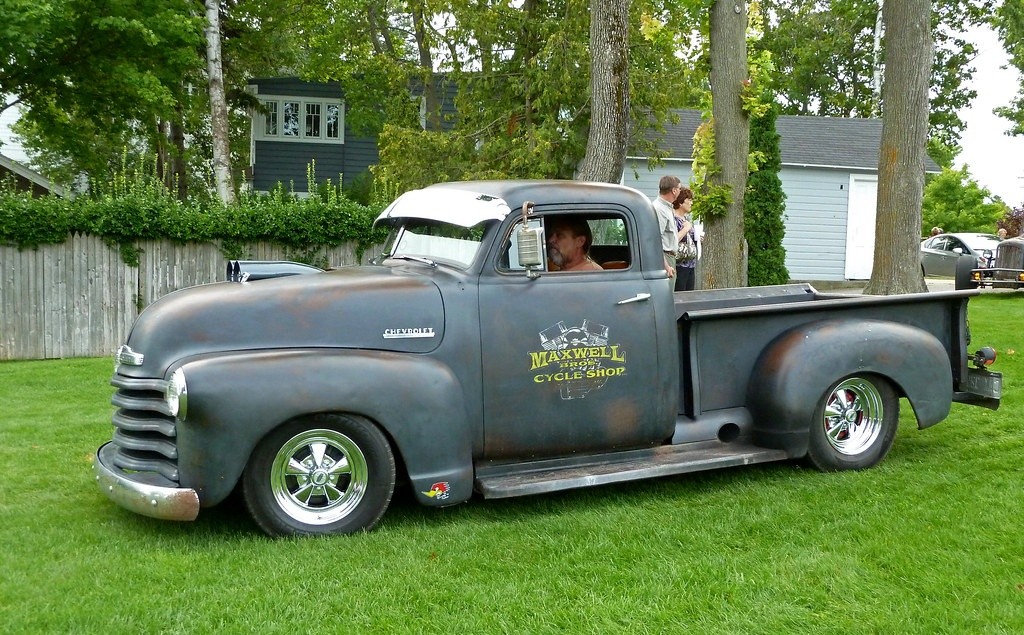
[95,179,1003,538]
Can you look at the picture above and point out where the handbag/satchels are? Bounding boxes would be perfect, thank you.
[676,242,697,262]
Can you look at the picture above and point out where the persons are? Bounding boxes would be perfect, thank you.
[652,176,682,292]
[544,215,606,271]
[672,186,704,292]
[931,227,943,245]
[997,228,1007,240]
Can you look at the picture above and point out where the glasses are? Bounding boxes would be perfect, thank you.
[675,186,682,191]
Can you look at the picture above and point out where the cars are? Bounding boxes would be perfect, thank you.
[922,229,1003,283]
